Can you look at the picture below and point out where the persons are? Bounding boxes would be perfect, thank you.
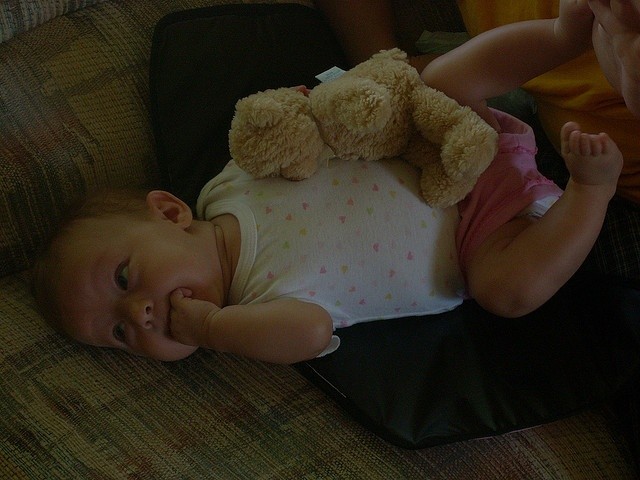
[34,0,626,367]
[229,47,501,209]
